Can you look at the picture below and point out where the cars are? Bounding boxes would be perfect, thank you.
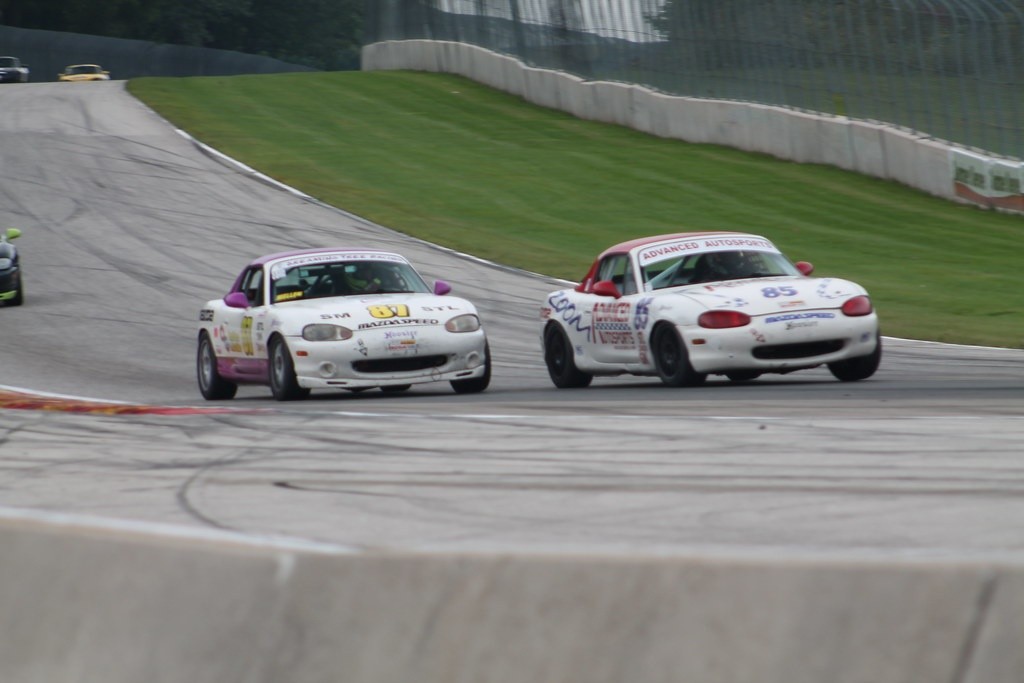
[540,231,883,388]
[0,228,23,306]
[0,56,30,84]
[195,247,493,403]
[57,65,111,81]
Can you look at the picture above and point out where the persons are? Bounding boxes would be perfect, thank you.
[707,252,742,279]
[336,263,385,296]
